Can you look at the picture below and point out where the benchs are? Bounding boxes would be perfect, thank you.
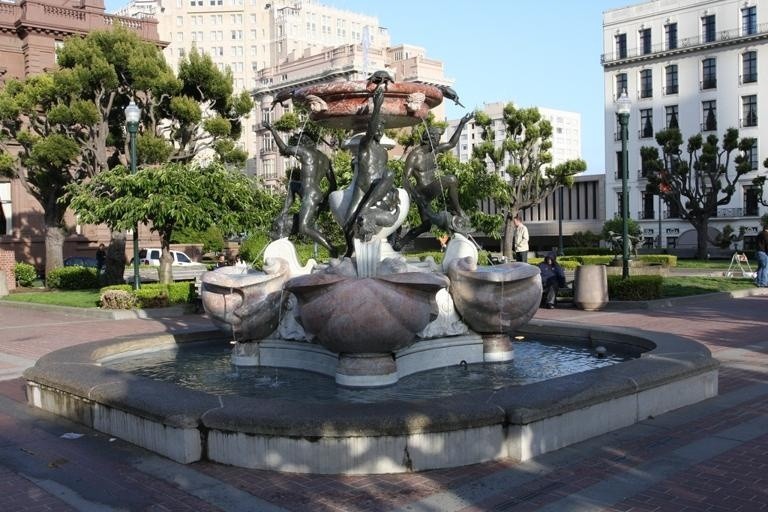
[556,267,574,308]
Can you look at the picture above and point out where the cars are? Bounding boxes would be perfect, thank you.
[63,256,107,270]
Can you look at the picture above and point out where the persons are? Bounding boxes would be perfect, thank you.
[513,216,530,265]
[754,224,768,288]
[539,248,566,310]
[343,84,400,259]
[391,108,475,252]
[233,253,246,264]
[96,243,106,275]
[216,254,228,268]
[259,118,346,261]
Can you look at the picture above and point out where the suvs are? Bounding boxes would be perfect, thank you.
[129,248,203,266]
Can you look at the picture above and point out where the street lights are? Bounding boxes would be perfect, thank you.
[615,88,632,279]
[124,96,142,290]
